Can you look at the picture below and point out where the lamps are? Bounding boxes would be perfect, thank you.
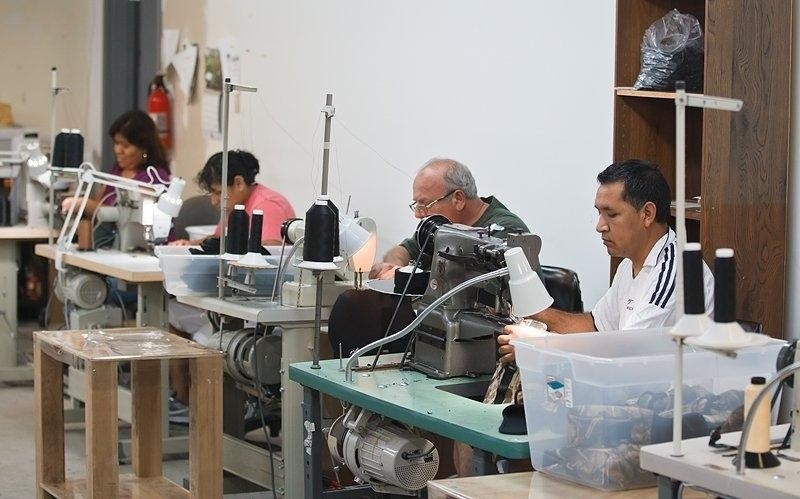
[275,214,372,305]
[345,248,554,384]
[1,141,50,177]
[53,164,186,272]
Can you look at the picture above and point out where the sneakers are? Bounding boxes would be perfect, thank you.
[167,399,190,422]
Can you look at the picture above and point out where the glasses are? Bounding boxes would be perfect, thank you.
[408,189,457,213]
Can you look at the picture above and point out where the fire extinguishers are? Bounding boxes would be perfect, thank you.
[148,74,170,150]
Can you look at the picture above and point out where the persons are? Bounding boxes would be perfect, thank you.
[60,110,177,322]
[496,157,715,364]
[166,149,296,424]
[368,157,531,279]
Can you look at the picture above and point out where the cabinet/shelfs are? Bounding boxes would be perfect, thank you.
[609,0,794,348]
[25,324,232,498]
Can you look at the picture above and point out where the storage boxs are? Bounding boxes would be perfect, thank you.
[498,326,788,494]
